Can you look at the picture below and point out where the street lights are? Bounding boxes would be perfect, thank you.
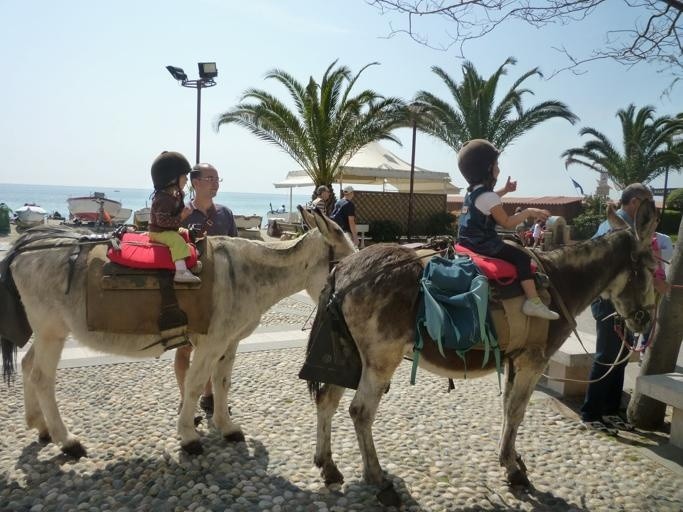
[165,61,219,235]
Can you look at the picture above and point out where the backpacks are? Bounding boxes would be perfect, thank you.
[410,245,501,393]
[330,201,351,231]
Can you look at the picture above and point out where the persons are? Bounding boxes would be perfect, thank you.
[276,204,285,212]
[334,186,360,245]
[458,139,561,320]
[581,184,672,437]
[175,164,238,424]
[310,186,331,216]
[513,206,545,250]
[147,152,202,283]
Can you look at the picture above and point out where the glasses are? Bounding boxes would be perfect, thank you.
[198,176,223,183]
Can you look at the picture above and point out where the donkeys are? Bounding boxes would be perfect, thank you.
[300,194,661,510]
[0,200,360,460]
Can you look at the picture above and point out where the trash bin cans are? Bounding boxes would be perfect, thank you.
[540,230,552,251]
[0,203,10,237]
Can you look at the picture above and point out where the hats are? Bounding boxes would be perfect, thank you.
[343,186,354,194]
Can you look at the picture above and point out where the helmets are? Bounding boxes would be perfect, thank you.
[457,139,500,184]
[151,151,191,187]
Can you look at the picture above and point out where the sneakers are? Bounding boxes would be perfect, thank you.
[177,394,231,424]
[522,300,560,320]
[580,416,636,436]
[173,269,201,283]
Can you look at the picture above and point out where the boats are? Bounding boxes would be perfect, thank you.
[14,202,48,227]
[232,213,263,231]
[66,190,151,228]
